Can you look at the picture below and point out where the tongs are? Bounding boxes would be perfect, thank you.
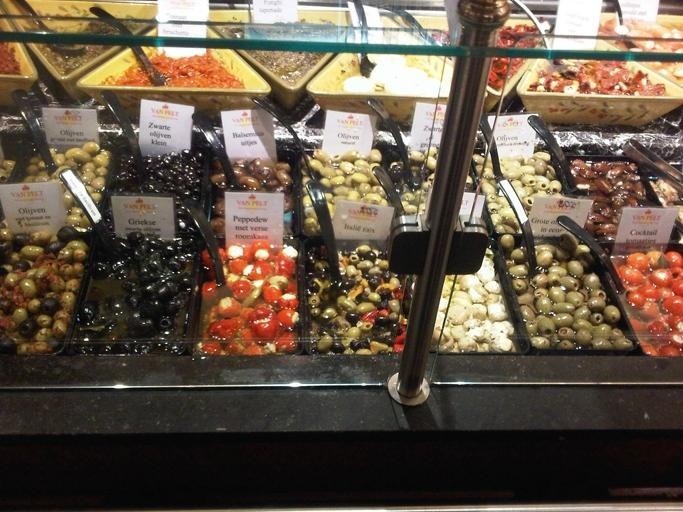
[623,138,683,195]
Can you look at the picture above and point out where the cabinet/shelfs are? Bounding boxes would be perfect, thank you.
[424,1,682,387]
[0,0,473,390]
